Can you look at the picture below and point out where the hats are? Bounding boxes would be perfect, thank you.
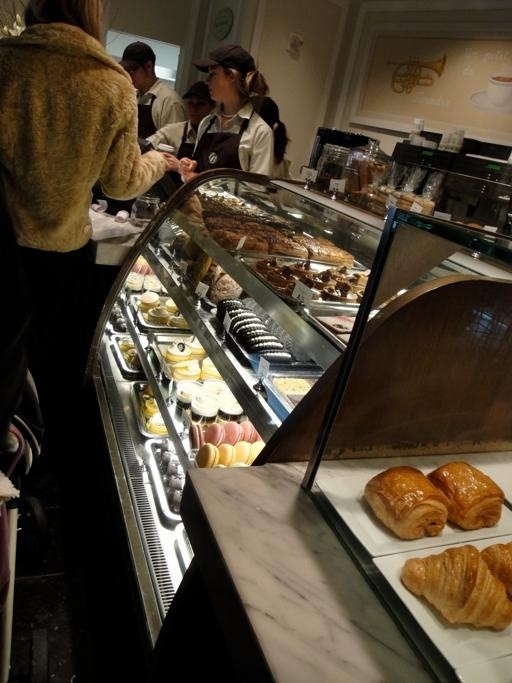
[118,40,156,72]
[182,81,210,101]
[192,43,254,73]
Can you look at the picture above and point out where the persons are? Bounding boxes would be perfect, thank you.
[148,82,217,160]
[1,0,180,455]
[252,95,290,182]
[178,45,272,183]
[118,42,185,150]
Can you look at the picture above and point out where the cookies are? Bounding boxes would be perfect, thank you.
[386,190,437,220]
[369,181,393,214]
[216,295,312,364]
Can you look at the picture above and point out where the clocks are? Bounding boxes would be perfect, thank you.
[212,7,233,41]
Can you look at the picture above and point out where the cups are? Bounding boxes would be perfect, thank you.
[414,118,424,135]
[487,75,512,107]
[157,143,175,154]
[409,134,424,146]
[423,140,434,149]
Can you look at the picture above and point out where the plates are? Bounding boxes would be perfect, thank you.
[110,334,144,376]
[129,380,168,438]
[173,261,189,282]
[227,251,367,271]
[144,438,182,523]
[469,89,509,114]
[151,331,191,380]
[189,281,218,311]
[261,370,326,422]
[159,243,175,261]
[219,320,322,371]
[129,294,194,334]
[237,258,356,305]
[302,303,381,351]
[107,322,125,335]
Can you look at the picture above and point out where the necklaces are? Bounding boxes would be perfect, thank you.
[219,107,238,120]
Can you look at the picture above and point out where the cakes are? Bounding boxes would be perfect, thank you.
[171,358,201,382]
[131,288,188,329]
[156,439,186,511]
[165,231,242,304]
[160,332,192,363]
[186,335,207,360]
[131,379,170,436]
[187,418,266,467]
[199,356,222,380]
[242,255,369,304]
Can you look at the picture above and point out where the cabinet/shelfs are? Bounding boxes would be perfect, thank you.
[83,168,512,650]
[301,207,512,683]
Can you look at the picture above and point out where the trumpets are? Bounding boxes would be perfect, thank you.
[385,55,448,95]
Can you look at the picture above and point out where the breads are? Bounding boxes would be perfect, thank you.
[398,545,512,633]
[482,540,512,598]
[190,188,355,265]
[428,460,505,531]
[119,340,139,366]
[362,464,452,543]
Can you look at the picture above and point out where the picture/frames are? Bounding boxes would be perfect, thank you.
[349,21,512,146]
